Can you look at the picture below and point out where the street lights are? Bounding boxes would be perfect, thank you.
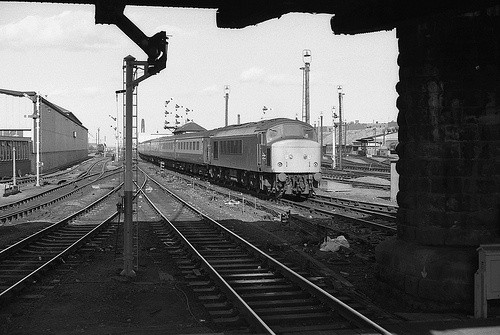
[224,85,230,126]
[320,112,323,166]
[337,85,342,171]
[302,49,311,123]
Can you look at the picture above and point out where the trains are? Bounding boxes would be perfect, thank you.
[137,118,321,202]
